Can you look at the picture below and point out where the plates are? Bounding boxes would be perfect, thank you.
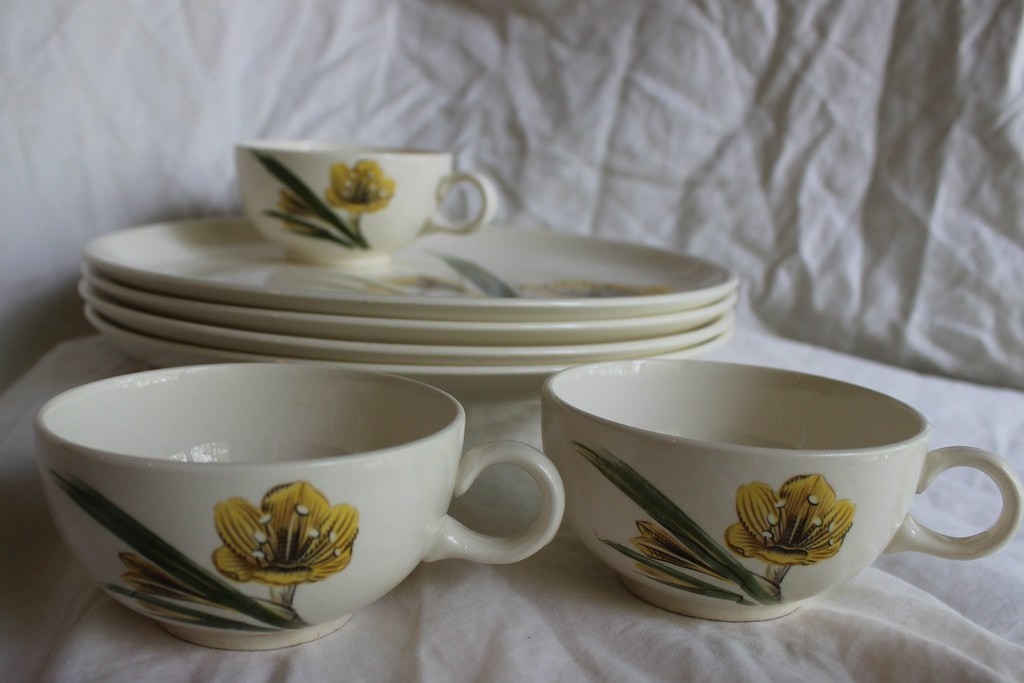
[78,216,740,378]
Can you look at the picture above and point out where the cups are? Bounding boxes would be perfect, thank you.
[540,360,1021,623]
[33,363,567,649]
[236,143,497,267]
